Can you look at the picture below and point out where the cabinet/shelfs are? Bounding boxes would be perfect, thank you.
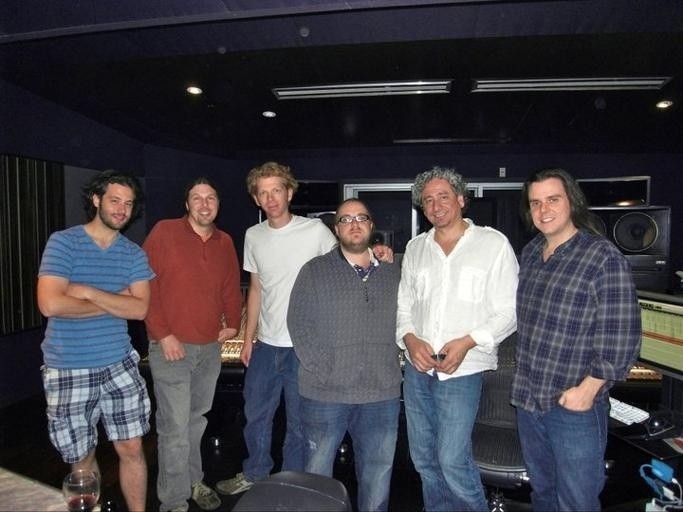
[101,495,119,512]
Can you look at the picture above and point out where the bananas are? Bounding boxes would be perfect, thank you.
[608,396,650,426]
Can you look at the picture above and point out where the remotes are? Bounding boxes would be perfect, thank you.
[649,415,672,433]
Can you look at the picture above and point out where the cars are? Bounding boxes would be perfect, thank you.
[60,466,100,512]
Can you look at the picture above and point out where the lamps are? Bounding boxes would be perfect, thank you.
[472,335,530,512]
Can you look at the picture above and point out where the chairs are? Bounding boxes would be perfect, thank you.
[215,471,252,497]
[190,481,222,510]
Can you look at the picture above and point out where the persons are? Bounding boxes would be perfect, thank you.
[395,166,519,512]
[36,171,157,512]
[509,172,642,512]
[284,198,403,512]
[216,164,393,512]
[137,176,242,511]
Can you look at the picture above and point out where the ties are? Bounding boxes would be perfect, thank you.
[633,290,682,417]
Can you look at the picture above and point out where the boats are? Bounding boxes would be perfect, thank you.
[580,205,673,256]
[307,211,337,238]
[367,230,395,250]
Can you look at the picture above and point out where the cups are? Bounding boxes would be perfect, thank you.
[336,213,372,226]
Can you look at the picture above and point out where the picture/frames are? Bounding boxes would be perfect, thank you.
[0,464,101,512]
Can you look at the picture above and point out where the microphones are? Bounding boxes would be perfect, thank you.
[232,470,348,511]
